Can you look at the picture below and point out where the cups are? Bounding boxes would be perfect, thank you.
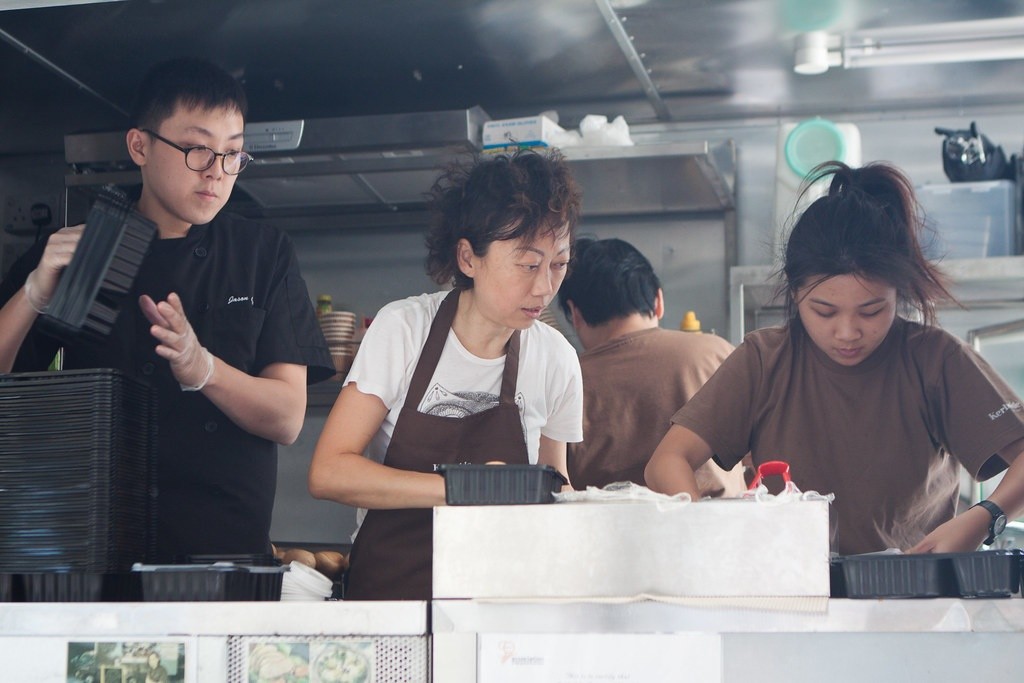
[317,312,356,356]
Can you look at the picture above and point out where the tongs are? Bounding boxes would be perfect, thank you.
[741,461,801,502]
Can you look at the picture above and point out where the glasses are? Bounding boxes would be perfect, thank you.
[141,128,254,176]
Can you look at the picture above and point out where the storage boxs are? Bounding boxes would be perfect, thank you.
[914,180,1021,261]
[482,116,577,157]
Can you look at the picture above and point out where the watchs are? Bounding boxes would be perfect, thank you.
[968,501,1008,545]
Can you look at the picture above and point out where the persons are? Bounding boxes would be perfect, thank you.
[307,141,584,600]
[0,56,338,602]
[554,235,749,496]
[644,160,1024,552]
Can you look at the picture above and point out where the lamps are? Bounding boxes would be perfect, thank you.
[793,34,1024,75]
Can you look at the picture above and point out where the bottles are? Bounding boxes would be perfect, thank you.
[680,311,702,334]
[315,294,332,313]
